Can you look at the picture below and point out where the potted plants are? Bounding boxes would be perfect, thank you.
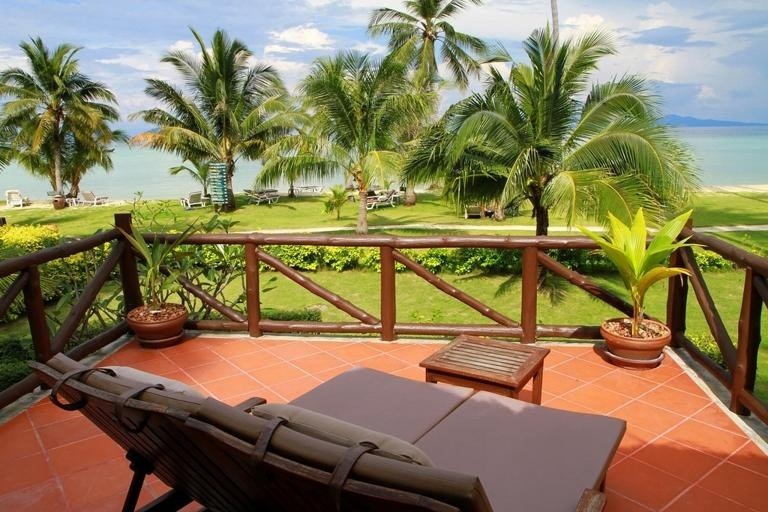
[110,214,203,348]
[577,204,709,371]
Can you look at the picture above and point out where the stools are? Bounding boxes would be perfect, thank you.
[419,332,551,405]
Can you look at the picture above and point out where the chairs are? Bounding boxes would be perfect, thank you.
[366,190,397,210]
[180,191,204,210]
[80,190,109,205]
[4,190,22,208]
[244,189,279,205]
